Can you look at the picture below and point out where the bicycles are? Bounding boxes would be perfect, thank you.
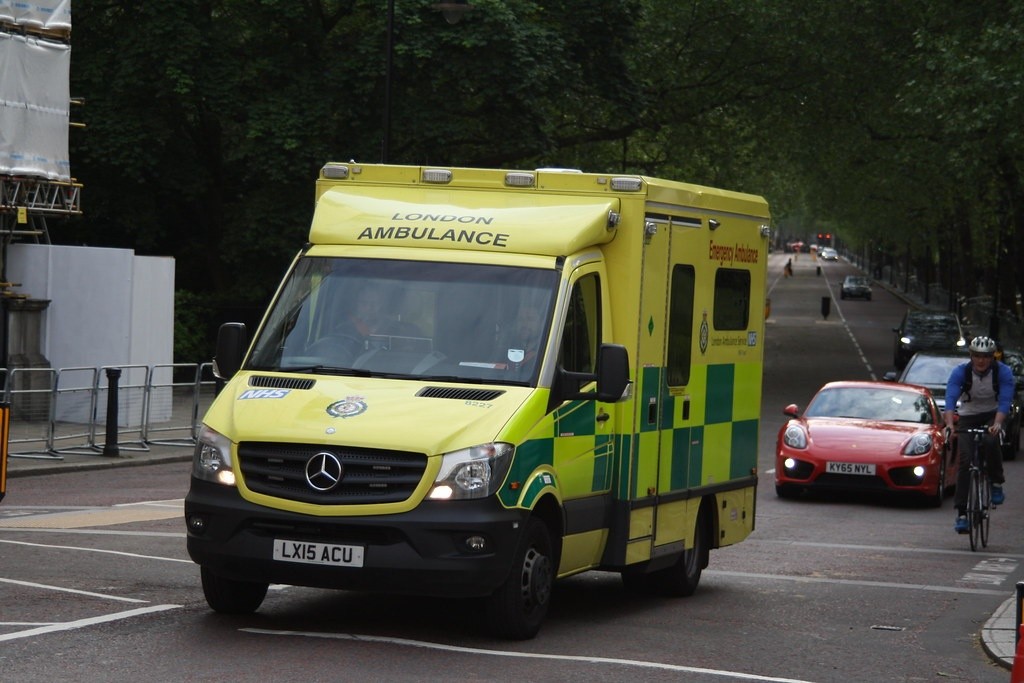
[945,426,1006,552]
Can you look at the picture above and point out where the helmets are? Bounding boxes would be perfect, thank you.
[968,335,998,358]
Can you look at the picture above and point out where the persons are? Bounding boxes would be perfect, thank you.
[338,270,421,340]
[942,336,1016,531]
[489,301,542,365]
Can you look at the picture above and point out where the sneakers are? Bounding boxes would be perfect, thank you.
[954,515,970,532]
[990,484,1005,504]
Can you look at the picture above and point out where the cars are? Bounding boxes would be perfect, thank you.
[777,380,963,511]
[839,275,873,301]
[817,246,838,261]
[883,351,971,419]
[892,307,968,371]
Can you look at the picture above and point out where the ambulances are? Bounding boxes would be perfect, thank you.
[185,167,770,647]
